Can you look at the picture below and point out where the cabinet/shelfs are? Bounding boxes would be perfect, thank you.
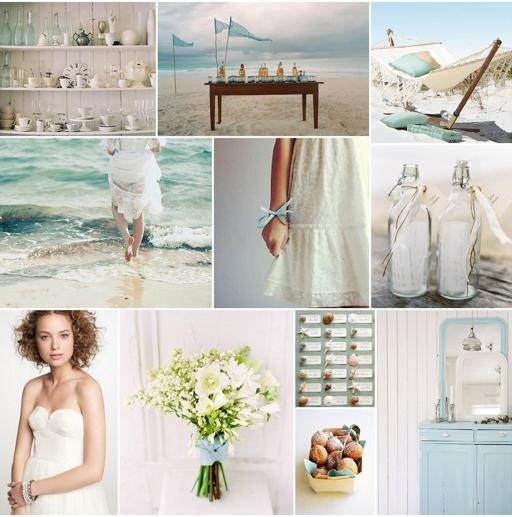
[0,2,156,137]
[417,417,512,514]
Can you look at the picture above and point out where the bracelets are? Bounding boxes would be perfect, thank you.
[19,478,38,506]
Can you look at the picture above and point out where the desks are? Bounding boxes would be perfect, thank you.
[206,76,322,128]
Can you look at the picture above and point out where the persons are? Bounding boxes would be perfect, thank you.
[98,138,168,263]
[7,310,107,515]
[214,137,369,308]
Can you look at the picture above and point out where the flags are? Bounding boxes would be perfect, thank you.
[173,34,194,48]
[228,20,273,42]
[215,20,229,34]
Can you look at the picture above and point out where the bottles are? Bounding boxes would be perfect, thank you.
[277,64,280,76]
[1,53,10,87]
[278,61,284,76]
[220,69,224,77]
[439,161,481,298]
[147,8,157,45]
[239,64,245,77]
[292,62,297,76]
[1,10,11,45]
[262,63,268,76]
[259,65,263,76]
[388,164,432,297]
[38,17,49,45]
[65,8,72,45]
[53,12,64,45]
[13,7,24,45]
[298,70,303,81]
[300,71,308,80]
[221,65,225,75]
[25,9,35,45]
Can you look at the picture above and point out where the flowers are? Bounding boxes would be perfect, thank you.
[126,343,278,502]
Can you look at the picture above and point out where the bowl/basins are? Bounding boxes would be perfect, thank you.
[50,125,59,130]
[127,113,143,127]
[101,114,115,124]
[77,106,91,118]
[19,117,32,127]
[66,124,78,130]
[1,120,15,130]
[100,126,117,132]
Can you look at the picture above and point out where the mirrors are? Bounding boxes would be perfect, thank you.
[438,318,508,420]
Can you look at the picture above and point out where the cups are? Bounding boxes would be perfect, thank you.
[28,78,41,89]
[43,78,54,87]
[119,80,128,88]
[10,68,15,88]
[77,75,83,86]
[17,69,23,87]
[104,34,115,45]
[37,120,49,132]
[98,21,106,46]
[60,78,69,89]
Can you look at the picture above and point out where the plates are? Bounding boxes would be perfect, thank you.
[307,427,360,494]
[48,129,62,132]
[65,130,80,131]
[127,127,143,131]
[15,127,29,132]
[70,118,97,132]
